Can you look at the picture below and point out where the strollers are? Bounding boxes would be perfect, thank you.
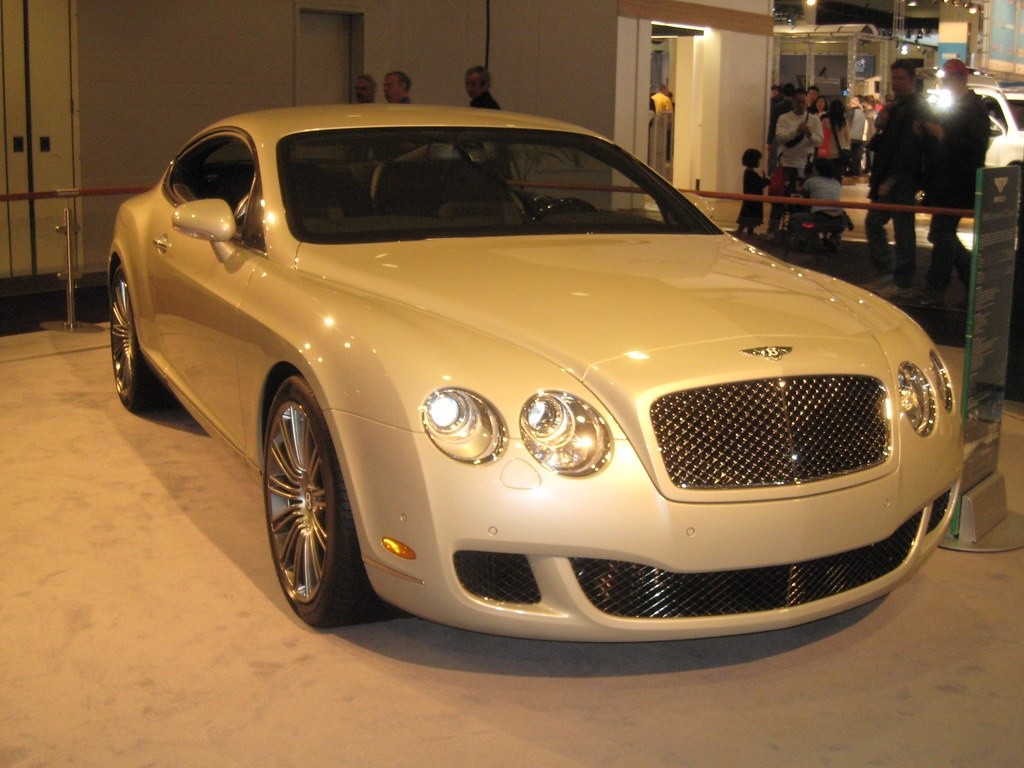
[777,151,829,251]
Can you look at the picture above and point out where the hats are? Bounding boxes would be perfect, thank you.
[944,59,976,74]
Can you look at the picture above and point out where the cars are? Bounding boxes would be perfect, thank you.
[109,103,965,641]
[914,67,1024,168]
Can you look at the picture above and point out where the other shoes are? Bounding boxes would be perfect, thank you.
[829,236,841,250]
[862,273,893,289]
[767,232,775,240]
[958,297,968,309]
[875,283,912,297]
[920,295,946,306]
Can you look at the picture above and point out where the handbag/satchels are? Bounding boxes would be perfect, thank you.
[768,165,786,196]
[840,149,861,176]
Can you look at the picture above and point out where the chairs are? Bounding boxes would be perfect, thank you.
[229,159,371,220]
[369,161,513,220]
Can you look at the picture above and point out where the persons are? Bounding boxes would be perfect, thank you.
[342,74,377,161]
[913,59,990,305]
[766,88,824,233]
[454,65,512,179]
[865,59,932,294]
[377,71,420,161]
[790,158,849,252]
[649,84,675,162]
[733,148,771,239]
[767,84,894,177]
